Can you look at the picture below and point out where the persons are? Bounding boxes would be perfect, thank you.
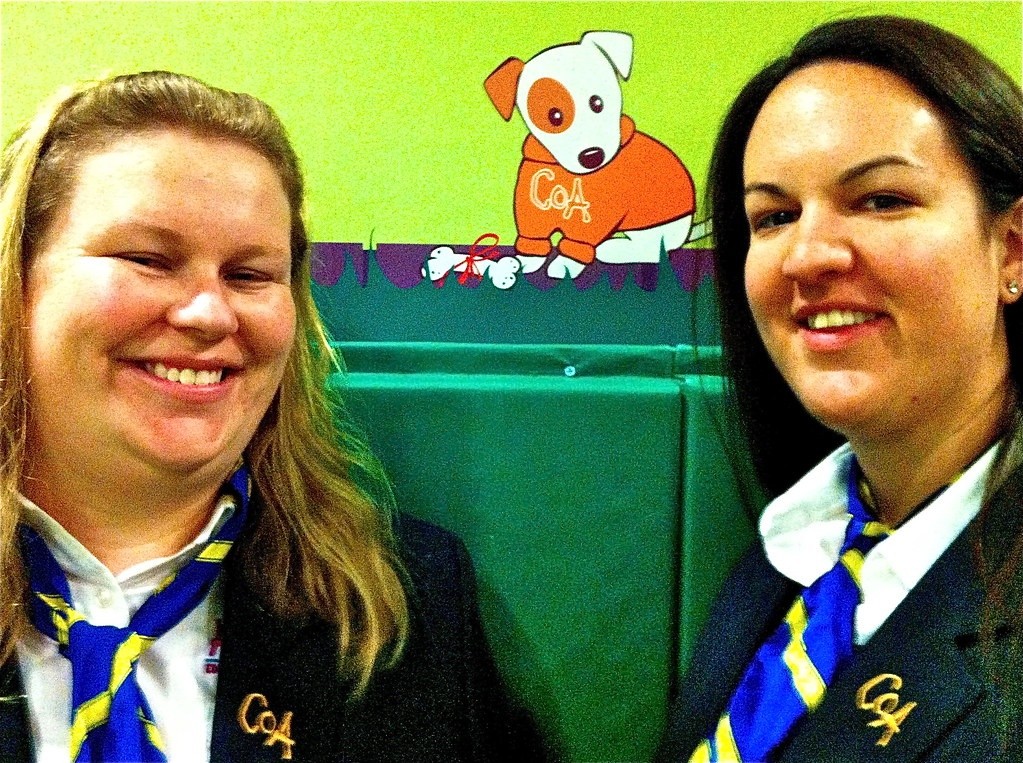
[649,16,1023,763]
[0,71,554,763]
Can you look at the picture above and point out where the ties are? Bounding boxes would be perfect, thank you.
[683,435,1002,763]
[16,464,253,763]
